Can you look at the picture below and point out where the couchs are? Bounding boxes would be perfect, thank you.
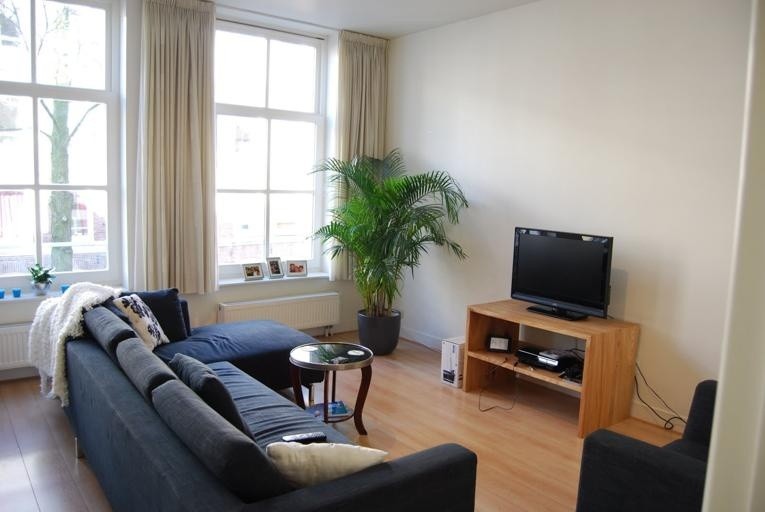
[58,296,479,511]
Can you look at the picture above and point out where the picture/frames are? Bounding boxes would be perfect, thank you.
[241,262,265,281]
[265,256,285,279]
[285,259,308,278]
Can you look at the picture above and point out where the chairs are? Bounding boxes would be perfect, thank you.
[573,375,719,512]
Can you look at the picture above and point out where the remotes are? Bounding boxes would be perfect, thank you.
[281,431,327,443]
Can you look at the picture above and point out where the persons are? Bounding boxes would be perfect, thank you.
[289,264,304,272]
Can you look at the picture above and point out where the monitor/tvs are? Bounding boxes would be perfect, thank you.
[511,226,614,321]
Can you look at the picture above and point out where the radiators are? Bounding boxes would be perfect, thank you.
[214,286,344,338]
[0,320,37,371]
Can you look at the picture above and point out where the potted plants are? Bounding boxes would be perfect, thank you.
[24,260,59,297]
[304,145,474,358]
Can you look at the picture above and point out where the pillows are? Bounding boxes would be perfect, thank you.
[104,297,131,324]
[118,286,190,342]
[112,292,171,354]
[265,440,392,490]
[164,347,258,444]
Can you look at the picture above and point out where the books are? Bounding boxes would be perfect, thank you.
[328,400,349,418]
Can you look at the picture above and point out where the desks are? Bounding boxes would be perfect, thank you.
[288,337,375,438]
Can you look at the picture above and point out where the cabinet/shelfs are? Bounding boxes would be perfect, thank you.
[462,295,645,441]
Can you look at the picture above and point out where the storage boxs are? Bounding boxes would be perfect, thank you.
[437,333,464,389]
